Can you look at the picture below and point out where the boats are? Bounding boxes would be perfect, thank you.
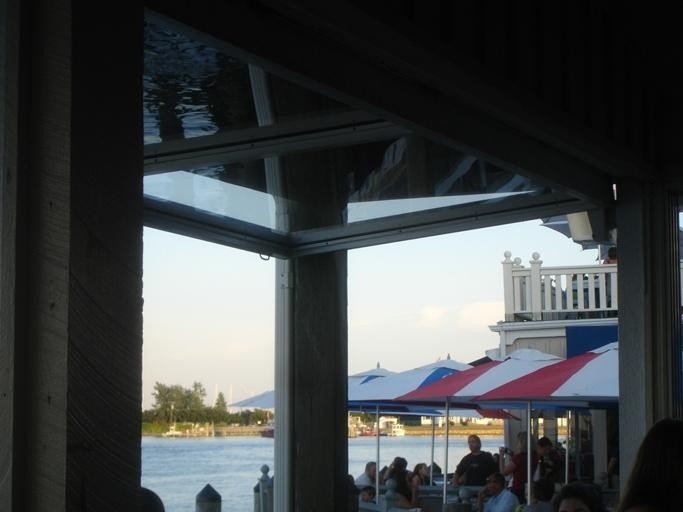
[159,414,405,438]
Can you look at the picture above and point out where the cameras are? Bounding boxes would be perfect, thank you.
[503,448,509,453]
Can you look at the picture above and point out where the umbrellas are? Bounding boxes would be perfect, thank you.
[540,214,607,264]
[392,348,618,505]
[225,388,274,411]
[348,360,441,505]
[468,340,620,406]
[347,353,525,505]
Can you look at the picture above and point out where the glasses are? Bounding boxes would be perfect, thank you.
[486,479,498,482]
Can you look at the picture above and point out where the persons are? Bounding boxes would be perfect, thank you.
[606,247,618,264]
[618,416,682,511]
[347,457,435,511]
[454,433,607,511]
